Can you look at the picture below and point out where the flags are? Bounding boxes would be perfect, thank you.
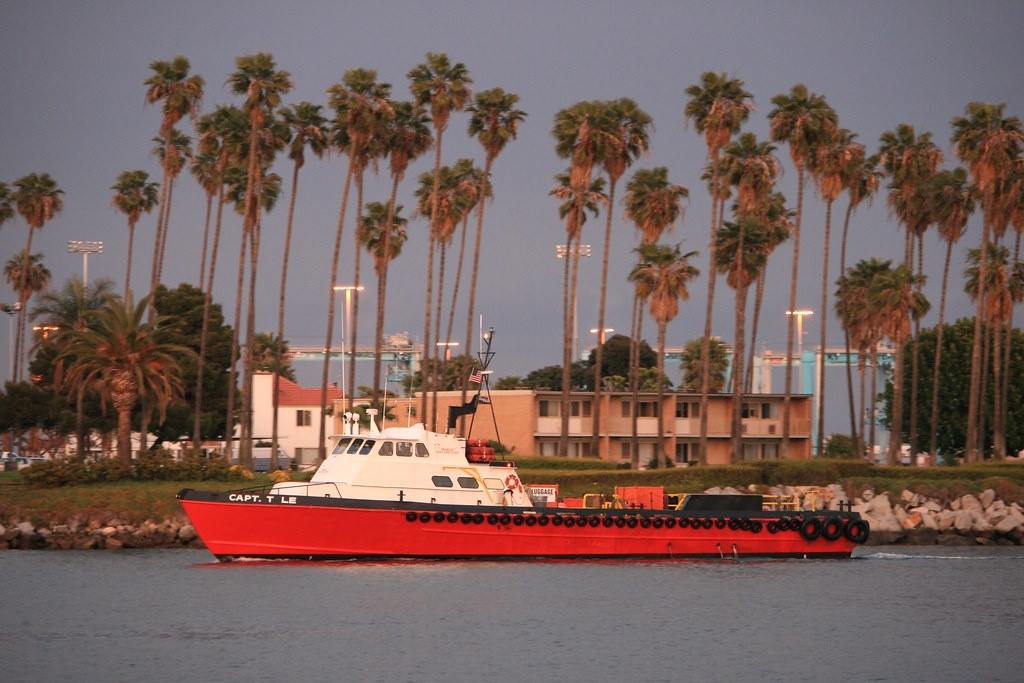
[469,367,485,384]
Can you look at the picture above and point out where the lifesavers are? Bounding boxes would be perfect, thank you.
[844,518,869,543]
[821,515,844,540]
[505,474,519,489]
[405,511,801,534]
[799,517,821,541]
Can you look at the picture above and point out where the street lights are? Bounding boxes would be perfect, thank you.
[786,308,815,394]
[67,240,103,300]
[0,301,21,382]
[591,328,614,345]
[436,342,460,361]
[335,285,365,355]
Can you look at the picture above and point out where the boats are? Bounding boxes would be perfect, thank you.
[174,307,876,566]
